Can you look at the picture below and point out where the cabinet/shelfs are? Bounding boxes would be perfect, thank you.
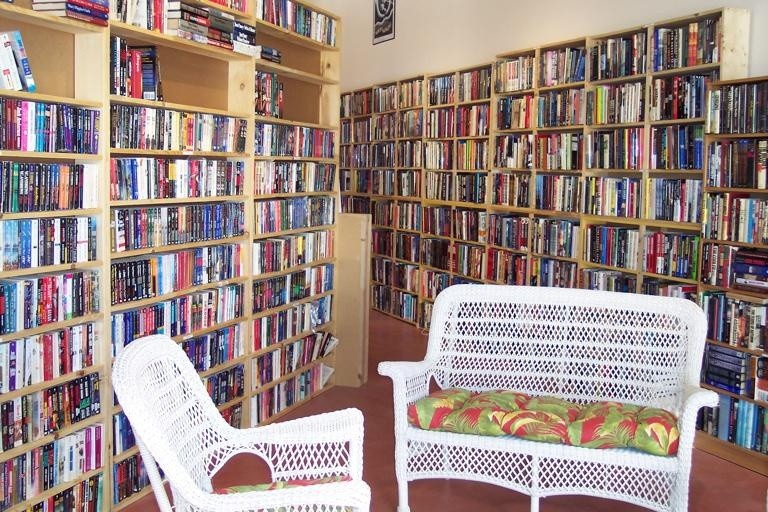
[1,2,767,512]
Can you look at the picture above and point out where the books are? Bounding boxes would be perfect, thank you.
[338,12,768,456]
[0,0,339,512]
